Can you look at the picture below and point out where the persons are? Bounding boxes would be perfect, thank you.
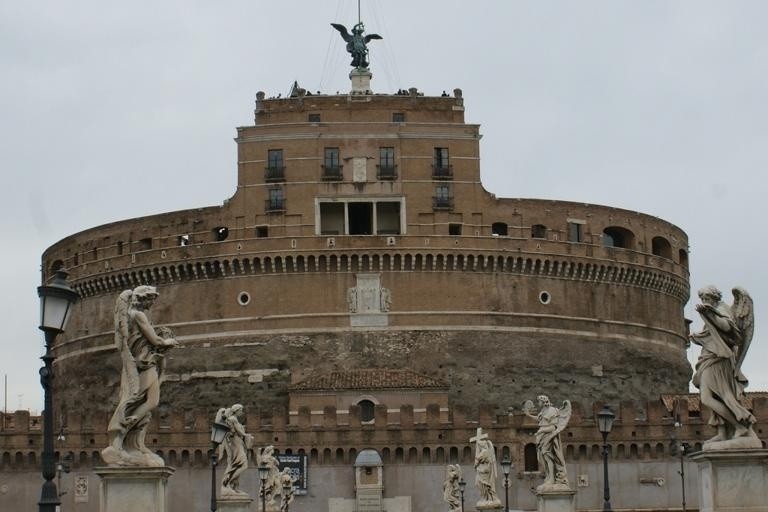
[101,286,177,467]
[260,446,280,496]
[213,404,248,498]
[524,395,572,484]
[688,288,758,439]
[472,440,501,502]
[443,464,461,512]
[353,32,366,67]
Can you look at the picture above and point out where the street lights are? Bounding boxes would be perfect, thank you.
[37,268,81,512]
[257,462,270,512]
[458,477,467,512]
[500,454,512,512]
[672,421,686,512]
[207,415,231,512]
[597,403,617,512]
[283,481,291,512]
